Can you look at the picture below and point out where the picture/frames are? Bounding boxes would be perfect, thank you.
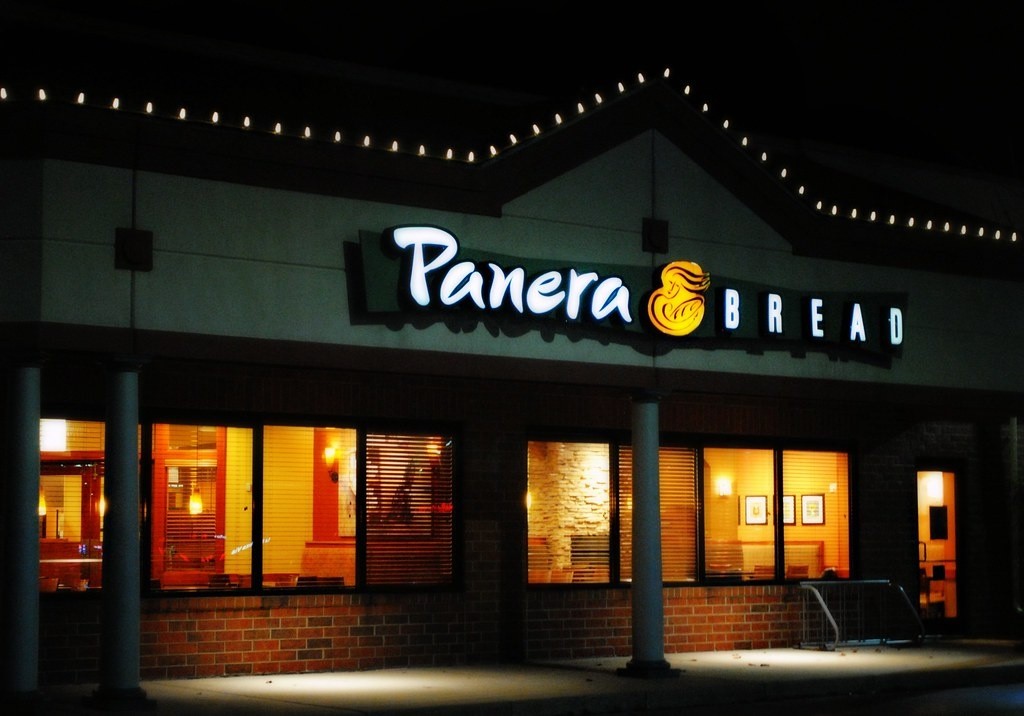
[745,495,768,525]
[801,493,825,525]
[773,495,796,525]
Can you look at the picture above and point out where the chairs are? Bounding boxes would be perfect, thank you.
[754,565,774,579]
[210,574,230,583]
[39,578,61,592]
[294,576,344,586]
[66,578,91,591]
[785,566,809,578]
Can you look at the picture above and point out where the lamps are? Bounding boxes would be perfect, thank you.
[426,444,440,475]
[325,447,338,483]
[39,485,47,516]
[188,426,203,515]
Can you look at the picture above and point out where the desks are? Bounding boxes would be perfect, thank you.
[690,571,759,581]
[162,584,240,588]
